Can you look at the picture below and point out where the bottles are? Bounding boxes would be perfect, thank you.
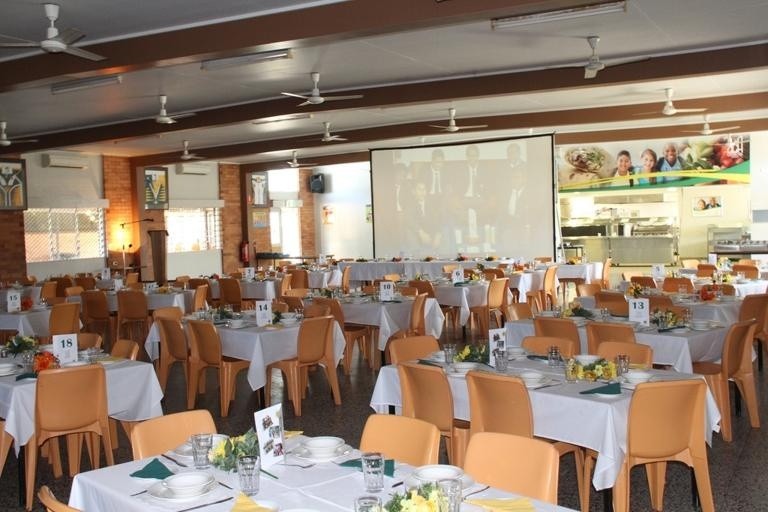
[607,218,612,236]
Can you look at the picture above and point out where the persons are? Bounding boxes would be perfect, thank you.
[706,197,721,210]
[392,143,528,253]
[251,177,266,205]
[675,140,702,170]
[656,143,691,183]
[145,173,167,204]
[694,200,706,210]
[607,150,640,186]
[631,149,665,184]
[0,166,22,186]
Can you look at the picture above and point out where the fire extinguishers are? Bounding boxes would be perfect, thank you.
[241,239,249,262]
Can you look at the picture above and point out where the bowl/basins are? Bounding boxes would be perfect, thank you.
[563,146,613,174]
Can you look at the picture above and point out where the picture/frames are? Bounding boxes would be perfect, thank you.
[251,171,269,208]
[143,166,169,211]
[0,156,29,212]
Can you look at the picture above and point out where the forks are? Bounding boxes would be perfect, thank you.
[276,461,316,470]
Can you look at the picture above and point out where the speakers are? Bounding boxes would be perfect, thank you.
[310,173,332,193]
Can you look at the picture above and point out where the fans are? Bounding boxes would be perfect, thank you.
[680,118,741,135]
[544,36,651,79]
[631,88,707,116]
[428,108,489,132]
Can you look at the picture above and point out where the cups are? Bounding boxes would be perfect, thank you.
[557,253,589,264]
[235,454,260,496]
[398,269,486,287]
[190,431,212,470]
[354,494,382,510]
[203,263,343,279]
[182,304,306,331]
[360,451,384,494]
[615,260,752,306]
[95,279,191,295]
[436,477,461,512]
[506,259,543,275]
[314,283,416,307]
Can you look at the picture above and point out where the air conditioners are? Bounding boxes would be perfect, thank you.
[175,162,212,175]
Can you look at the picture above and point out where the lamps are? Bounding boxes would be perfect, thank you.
[378,98,454,111]
[490,0,628,29]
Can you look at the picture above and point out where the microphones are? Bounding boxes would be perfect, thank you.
[145,218,154,222]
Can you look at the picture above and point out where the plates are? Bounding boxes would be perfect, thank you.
[404,473,475,488]
[411,462,465,484]
[185,435,230,449]
[293,445,353,463]
[413,340,655,397]
[160,471,215,496]
[174,443,214,457]
[526,303,721,336]
[13,297,49,316]
[146,480,219,503]
[0,343,117,381]
[299,435,345,455]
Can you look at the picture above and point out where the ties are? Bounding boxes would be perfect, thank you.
[471,165,476,195]
[515,190,519,203]
[392,186,398,212]
[434,172,439,196]
[418,203,423,217]
